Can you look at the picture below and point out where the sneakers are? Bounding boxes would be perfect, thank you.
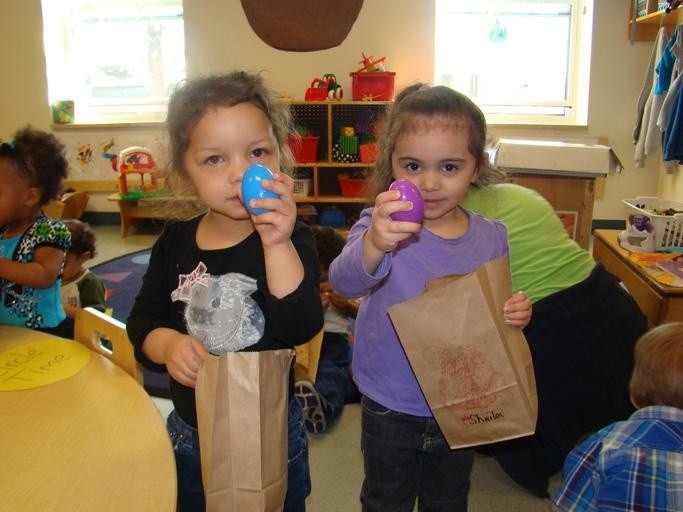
[293,380,335,436]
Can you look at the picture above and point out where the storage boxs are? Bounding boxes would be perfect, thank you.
[350,70,396,102]
[321,206,346,228]
[339,177,373,196]
[283,130,322,163]
[294,213,317,224]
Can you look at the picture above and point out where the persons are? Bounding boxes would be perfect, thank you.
[455,179,650,499]
[55,217,106,343]
[292,225,361,437]
[121,68,324,512]
[544,319,682,510]
[0,122,73,343]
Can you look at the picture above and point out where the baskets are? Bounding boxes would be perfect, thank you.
[622,195,683,253]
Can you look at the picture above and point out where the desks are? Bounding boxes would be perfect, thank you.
[593,227,682,340]
[0,320,183,512]
[105,191,209,239]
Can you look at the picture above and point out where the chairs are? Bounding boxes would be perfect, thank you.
[74,305,148,384]
[41,198,62,218]
[60,194,89,219]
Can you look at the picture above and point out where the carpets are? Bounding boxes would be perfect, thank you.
[82,248,172,400]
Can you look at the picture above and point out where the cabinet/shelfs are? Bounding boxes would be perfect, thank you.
[264,100,393,232]
